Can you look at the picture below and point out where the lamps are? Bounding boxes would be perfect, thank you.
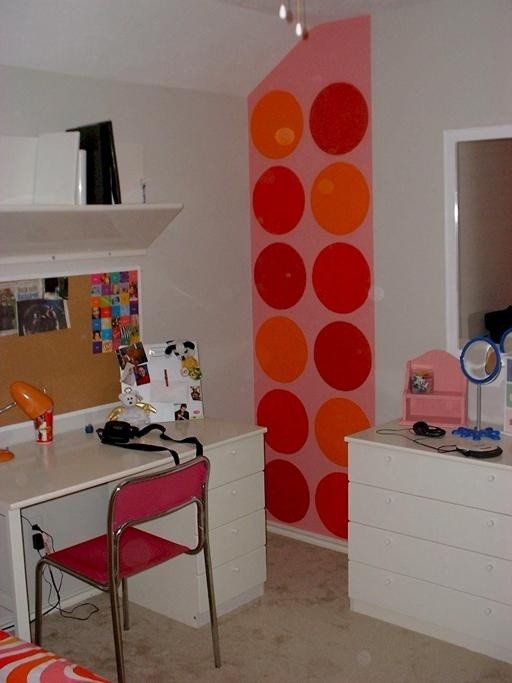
[0,379,54,463]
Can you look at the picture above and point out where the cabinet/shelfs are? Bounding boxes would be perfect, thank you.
[398,350,470,429]
[345,418,512,666]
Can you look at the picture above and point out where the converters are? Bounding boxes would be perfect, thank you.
[32,533,44,550]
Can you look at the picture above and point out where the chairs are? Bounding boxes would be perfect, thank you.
[32,459,225,681]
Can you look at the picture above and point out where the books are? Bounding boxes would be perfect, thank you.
[64,120,123,205]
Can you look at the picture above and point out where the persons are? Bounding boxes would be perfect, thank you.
[92,306,101,318]
[137,365,150,386]
[191,387,201,400]
[175,404,189,421]
[33,414,48,441]
[120,352,137,370]
[0,293,15,330]
[93,331,102,341]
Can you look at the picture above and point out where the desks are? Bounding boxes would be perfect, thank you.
[0,417,270,652]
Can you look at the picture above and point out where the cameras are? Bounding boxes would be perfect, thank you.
[102,421,133,443]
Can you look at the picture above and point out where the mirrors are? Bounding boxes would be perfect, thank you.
[443,125,512,361]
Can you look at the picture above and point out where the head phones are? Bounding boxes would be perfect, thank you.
[413,422,446,437]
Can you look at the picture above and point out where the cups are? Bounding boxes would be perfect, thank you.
[35,406,53,444]
